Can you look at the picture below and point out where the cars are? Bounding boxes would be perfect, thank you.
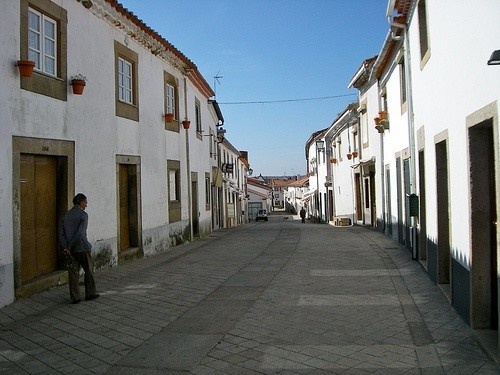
[255,209,268,221]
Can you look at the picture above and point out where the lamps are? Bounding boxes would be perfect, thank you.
[316,138,330,157]
[216,127,226,144]
[487,49,500,65]
[247,168,253,176]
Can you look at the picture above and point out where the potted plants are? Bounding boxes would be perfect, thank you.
[71,72,87,94]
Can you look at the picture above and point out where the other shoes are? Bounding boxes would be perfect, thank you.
[72,298,81,304]
[85,293,100,300]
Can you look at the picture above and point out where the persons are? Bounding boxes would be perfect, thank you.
[61,192,100,304]
[300,207,306,223]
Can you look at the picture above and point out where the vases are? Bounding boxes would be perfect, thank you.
[374,117,382,125]
[18,60,36,77]
[183,121,190,129]
[379,111,387,118]
[310,150,358,176]
[165,113,174,122]
